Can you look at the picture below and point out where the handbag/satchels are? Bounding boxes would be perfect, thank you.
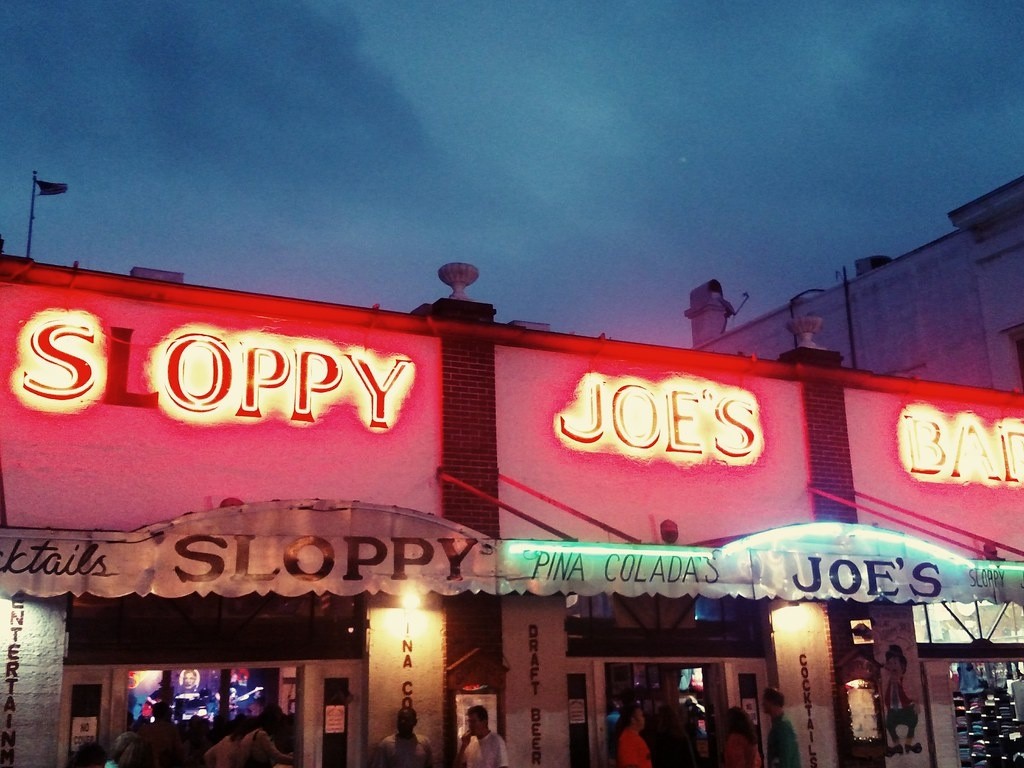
[244,731,273,768]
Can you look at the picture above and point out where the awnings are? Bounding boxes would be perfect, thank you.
[0,499,1024,606]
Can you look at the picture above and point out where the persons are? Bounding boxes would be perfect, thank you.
[760,687,803,768]
[128,686,295,768]
[722,705,762,768]
[375,707,434,768]
[106,731,153,768]
[608,688,717,768]
[457,705,509,768]
[66,742,107,768]
